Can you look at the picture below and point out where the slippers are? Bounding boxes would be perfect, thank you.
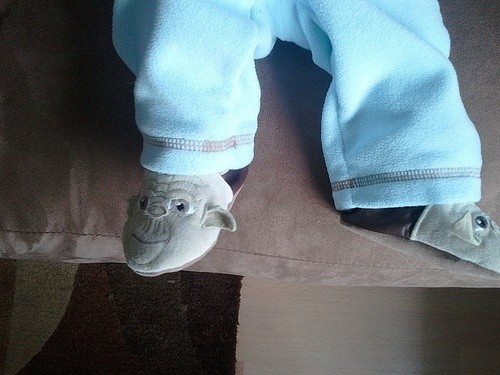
[342,203,500,273]
[121,164,250,276]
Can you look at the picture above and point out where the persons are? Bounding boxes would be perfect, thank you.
[111,0,499,283]
